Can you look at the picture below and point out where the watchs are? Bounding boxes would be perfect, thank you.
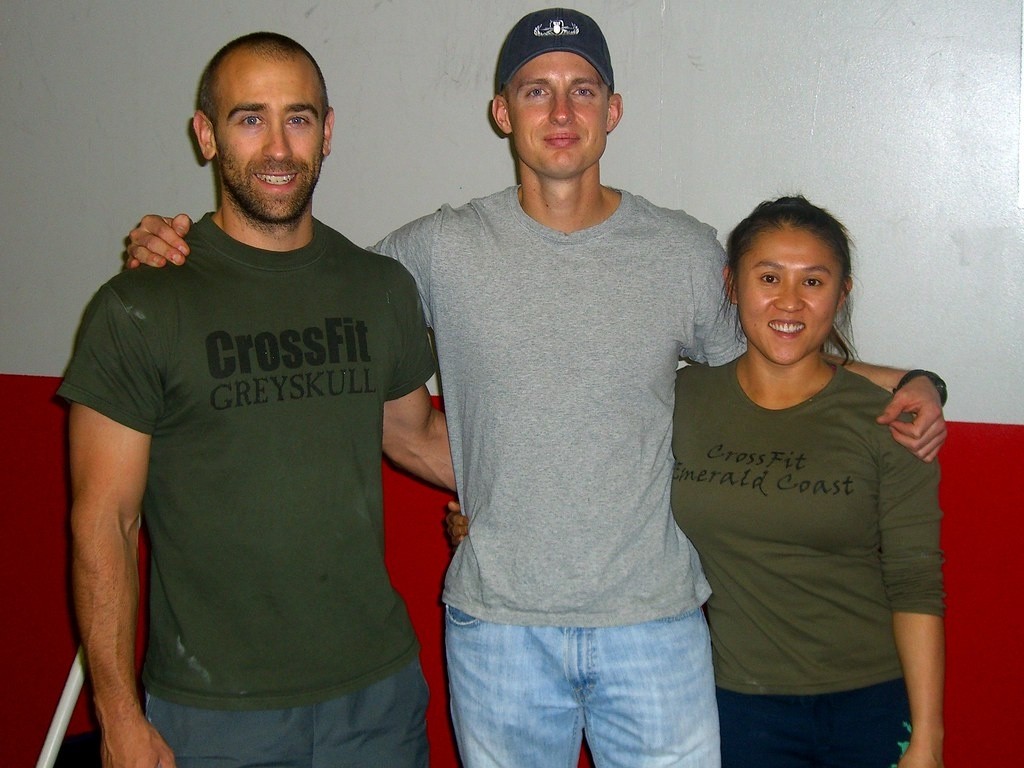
[888,366,948,409]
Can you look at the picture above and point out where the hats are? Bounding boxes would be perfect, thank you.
[499,9,614,86]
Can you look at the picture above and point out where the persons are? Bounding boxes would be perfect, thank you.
[125,6,948,768]
[441,193,947,768]
[52,32,457,768]
